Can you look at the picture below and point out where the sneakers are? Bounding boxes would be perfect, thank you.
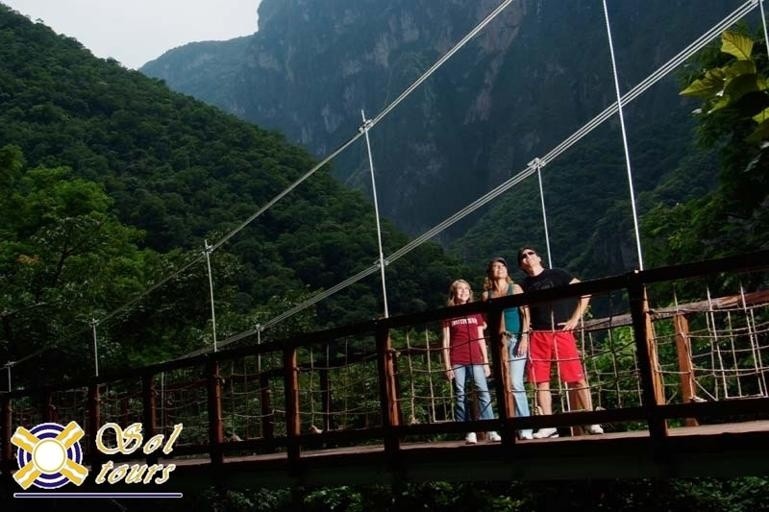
[462,424,605,445]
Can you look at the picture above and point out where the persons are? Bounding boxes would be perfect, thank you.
[480,256,535,440]
[513,246,605,439]
[441,278,502,444]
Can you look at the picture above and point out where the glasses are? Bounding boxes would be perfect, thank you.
[522,252,535,259]
